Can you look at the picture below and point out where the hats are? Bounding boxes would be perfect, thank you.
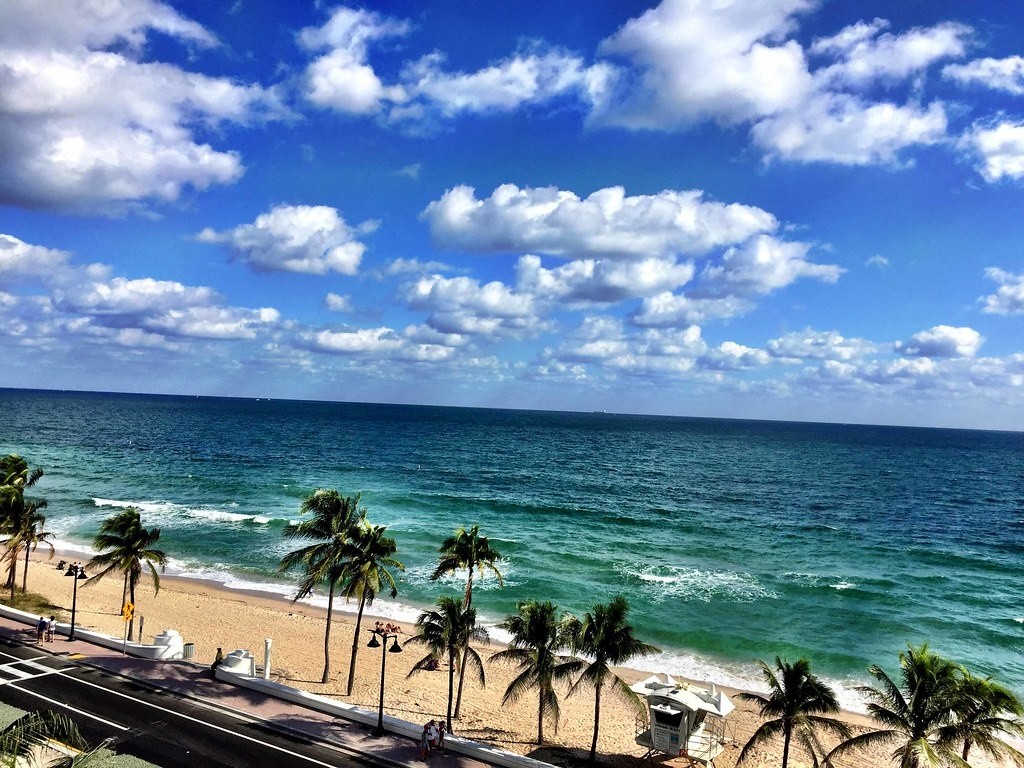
[424,724,430,729]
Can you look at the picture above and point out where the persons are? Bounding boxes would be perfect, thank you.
[56,560,83,572]
[438,720,446,747]
[36,617,46,646]
[375,620,402,633]
[428,720,438,751]
[420,724,430,762]
[211,648,223,670]
[47,616,56,643]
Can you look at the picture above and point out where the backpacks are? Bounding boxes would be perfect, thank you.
[39,621,46,631]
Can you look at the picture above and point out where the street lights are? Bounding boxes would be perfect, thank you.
[367,621,403,737]
[55,559,88,642]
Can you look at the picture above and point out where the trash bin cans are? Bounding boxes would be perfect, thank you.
[184,644,194,657]
